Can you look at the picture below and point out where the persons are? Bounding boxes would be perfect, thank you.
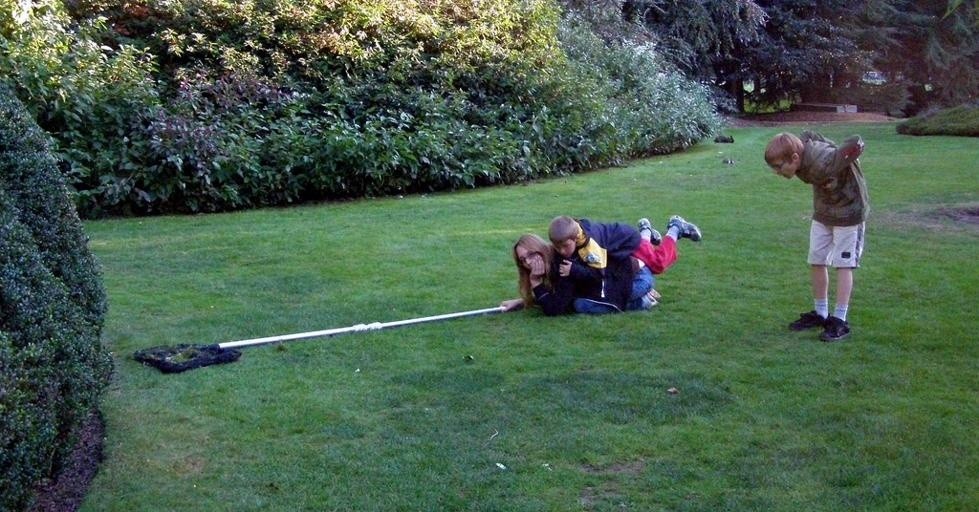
[514,234,661,316]
[547,215,701,287]
[764,130,871,341]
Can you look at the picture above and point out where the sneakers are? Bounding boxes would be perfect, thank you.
[637,218,662,245]
[668,215,702,240]
[788,309,830,331]
[818,316,850,341]
